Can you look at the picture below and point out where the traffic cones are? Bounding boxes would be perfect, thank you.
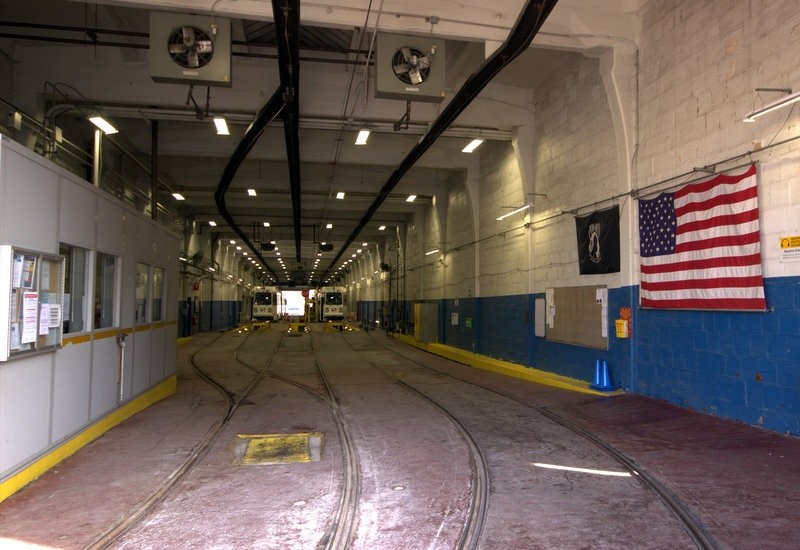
[590,359,617,391]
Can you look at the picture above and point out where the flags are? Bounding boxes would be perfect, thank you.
[576,204,620,275]
[636,164,767,313]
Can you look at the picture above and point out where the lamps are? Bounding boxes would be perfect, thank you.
[743,87,800,122]
[425,241,450,256]
[496,193,547,221]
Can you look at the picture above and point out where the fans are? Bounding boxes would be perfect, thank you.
[189,250,204,266]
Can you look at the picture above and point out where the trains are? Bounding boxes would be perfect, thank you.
[312,286,346,322]
[253,285,287,323]
